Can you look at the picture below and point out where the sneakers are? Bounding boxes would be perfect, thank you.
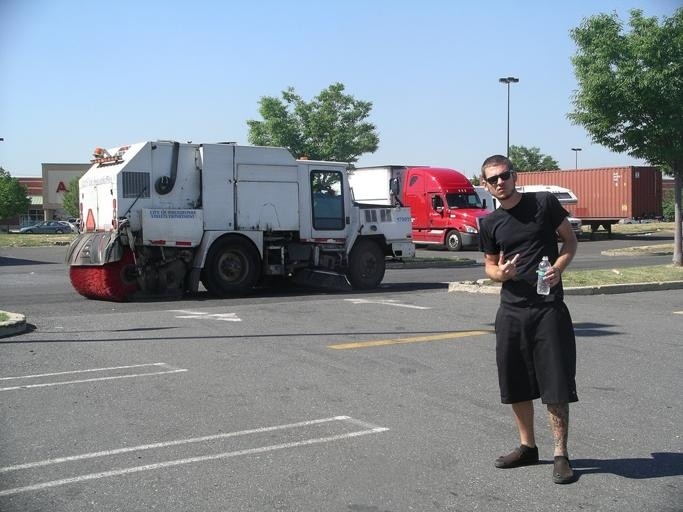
[552,455,575,485]
[494,445,539,468]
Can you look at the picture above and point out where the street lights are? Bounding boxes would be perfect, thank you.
[570,148,582,169]
[498,76,519,159]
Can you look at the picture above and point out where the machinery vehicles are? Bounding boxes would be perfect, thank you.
[65,140,415,302]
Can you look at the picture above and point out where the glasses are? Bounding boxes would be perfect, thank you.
[485,170,513,185]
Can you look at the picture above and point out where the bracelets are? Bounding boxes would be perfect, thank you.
[553,265,562,276]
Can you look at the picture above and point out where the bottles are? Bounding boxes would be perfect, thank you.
[536,256,551,295]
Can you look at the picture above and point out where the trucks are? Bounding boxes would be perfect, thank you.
[472,185,583,242]
[313,165,494,252]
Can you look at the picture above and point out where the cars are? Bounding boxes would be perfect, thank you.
[619,218,646,224]
[21,218,80,234]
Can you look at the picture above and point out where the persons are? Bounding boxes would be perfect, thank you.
[480,155,578,484]
